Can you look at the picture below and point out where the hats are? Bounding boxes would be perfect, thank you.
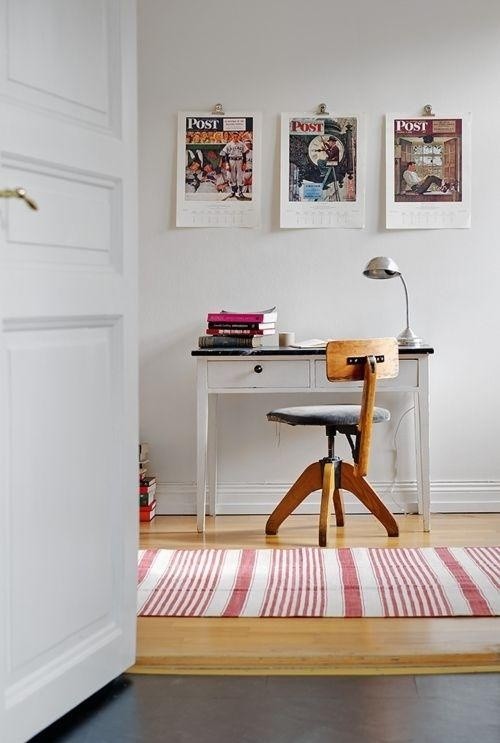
[232,131,239,136]
[327,136,337,142]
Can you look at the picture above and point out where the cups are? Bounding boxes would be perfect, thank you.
[279,332,296,346]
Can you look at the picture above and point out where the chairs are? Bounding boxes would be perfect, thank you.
[265,336,400,546]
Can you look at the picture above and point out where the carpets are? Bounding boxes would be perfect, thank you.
[136,544,500,619]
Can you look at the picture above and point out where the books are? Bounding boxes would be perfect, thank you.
[196,301,280,348]
[138,442,158,524]
[287,336,341,348]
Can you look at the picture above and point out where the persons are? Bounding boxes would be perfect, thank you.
[184,116,462,203]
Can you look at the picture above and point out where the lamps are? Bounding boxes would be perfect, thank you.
[362,254,425,348]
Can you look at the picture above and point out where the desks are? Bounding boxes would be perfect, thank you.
[190,344,436,533]
